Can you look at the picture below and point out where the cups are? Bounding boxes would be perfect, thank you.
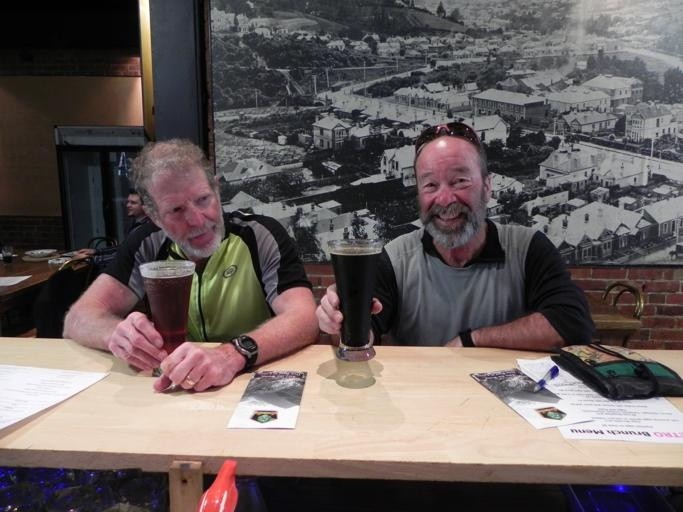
[137,260,196,377]
[327,239,383,362]
[1,246,13,264]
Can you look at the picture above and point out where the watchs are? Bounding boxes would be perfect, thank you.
[226,334,258,374]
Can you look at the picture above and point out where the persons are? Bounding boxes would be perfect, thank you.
[61,136,321,392]
[71,187,151,265]
[315,121,601,354]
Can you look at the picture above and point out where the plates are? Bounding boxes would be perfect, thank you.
[24,249,57,257]
[0,254,18,257]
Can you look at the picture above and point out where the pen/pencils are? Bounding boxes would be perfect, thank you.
[534,365,559,393]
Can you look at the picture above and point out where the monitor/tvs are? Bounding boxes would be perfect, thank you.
[52,120,144,252]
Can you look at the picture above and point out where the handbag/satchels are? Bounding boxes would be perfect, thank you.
[559,344,683,400]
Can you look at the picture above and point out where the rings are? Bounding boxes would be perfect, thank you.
[185,375,196,387]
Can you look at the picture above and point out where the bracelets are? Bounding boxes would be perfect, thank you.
[457,327,476,348]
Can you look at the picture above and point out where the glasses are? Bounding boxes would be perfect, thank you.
[414,122,481,155]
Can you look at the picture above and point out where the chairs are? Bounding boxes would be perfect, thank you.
[602,282,644,347]
[11,254,95,337]
[86,236,118,252]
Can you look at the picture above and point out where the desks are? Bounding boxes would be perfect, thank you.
[0,336,683,511]
[584,289,642,339]
[0,251,96,302]
